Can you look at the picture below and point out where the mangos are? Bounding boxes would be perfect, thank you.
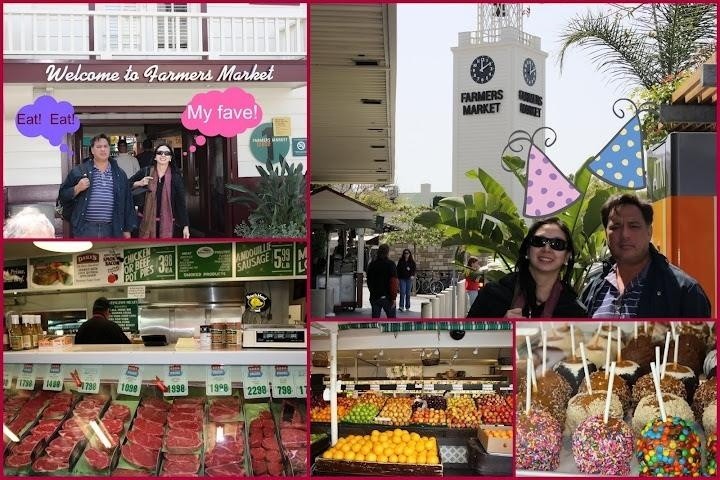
[484,428,513,439]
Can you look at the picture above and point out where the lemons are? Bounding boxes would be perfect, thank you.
[322,429,440,465]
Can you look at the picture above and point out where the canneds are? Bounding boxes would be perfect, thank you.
[199,317,242,350]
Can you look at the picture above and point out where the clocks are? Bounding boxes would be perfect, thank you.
[470,54,496,84]
[522,56,538,88]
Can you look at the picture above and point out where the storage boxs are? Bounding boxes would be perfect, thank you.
[476,423,513,454]
[313,435,443,476]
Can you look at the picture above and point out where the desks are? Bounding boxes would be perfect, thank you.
[465,436,512,477]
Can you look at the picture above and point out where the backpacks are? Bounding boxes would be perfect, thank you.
[54,164,90,220]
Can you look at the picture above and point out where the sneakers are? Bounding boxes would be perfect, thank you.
[398,306,411,312]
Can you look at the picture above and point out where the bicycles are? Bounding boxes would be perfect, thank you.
[414,269,453,296]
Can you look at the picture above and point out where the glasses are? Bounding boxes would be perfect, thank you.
[156,150,172,157]
[530,232,570,252]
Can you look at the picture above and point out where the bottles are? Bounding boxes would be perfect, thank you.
[199,317,241,351]
[6,314,45,350]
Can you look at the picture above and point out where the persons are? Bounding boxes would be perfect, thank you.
[135,140,155,166]
[52,134,136,240]
[112,140,140,178]
[396,248,417,312]
[126,144,192,239]
[575,194,712,319]
[75,297,132,344]
[466,257,480,309]
[468,217,589,318]
[366,243,399,318]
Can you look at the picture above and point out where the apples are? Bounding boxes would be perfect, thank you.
[309,392,513,429]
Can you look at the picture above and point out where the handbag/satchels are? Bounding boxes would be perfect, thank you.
[131,186,152,222]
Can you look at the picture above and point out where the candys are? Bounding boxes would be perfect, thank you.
[516,320,718,478]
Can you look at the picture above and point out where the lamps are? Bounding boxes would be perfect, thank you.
[377,345,480,360]
